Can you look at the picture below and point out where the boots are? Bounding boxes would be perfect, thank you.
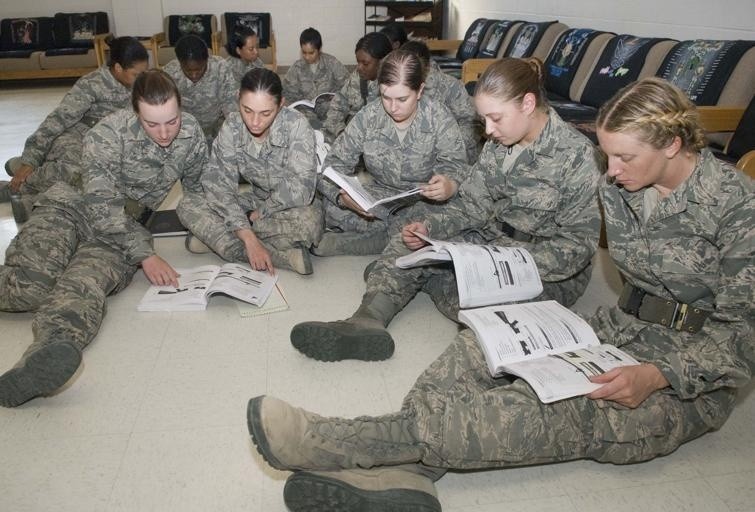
[290,290,402,362]
[310,228,391,257]
[245,394,422,473]
[242,243,313,276]
[0,324,89,408]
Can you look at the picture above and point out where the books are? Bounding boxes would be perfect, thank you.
[396,230,543,308]
[137,264,278,312]
[323,166,424,222]
[459,299,639,403]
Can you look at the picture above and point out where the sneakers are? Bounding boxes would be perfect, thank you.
[184,232,214,254]
[10,191,42,223]
[4,155,22,177]
[0,180,13,203]
[283,460,443,512]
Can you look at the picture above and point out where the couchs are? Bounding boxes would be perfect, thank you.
[0,12,112,79]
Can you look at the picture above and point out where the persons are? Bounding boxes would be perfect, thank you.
[163,27,489,166]
[311,50,469,256]
[0,68,210,407]
[176,68,326,276]
[0,36,149,222]
[246,77,755,512]
[289,58,607,362]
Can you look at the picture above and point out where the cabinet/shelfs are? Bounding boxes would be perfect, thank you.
[364,0,443,55]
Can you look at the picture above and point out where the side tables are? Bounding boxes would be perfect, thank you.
[101,33,154,68]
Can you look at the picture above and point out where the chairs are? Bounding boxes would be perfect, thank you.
[215,12,276,72]
[154,14,217,67]
